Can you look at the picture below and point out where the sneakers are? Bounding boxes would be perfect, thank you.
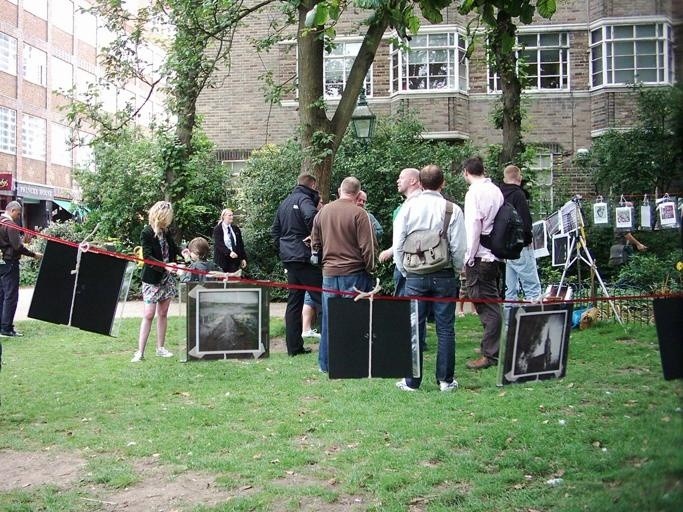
[458,312,478,318]
[289,345,312,356]
[302,328,320,338]
[130,346,173,361]
[397,378,460,392]
[466,357,498,369]
[0,329,24,338]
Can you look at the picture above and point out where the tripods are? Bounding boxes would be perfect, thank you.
[554,206,623,332]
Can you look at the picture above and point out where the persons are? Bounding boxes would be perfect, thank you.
[310,177,384,373]
[270,173,323,355]
[131,200,191,363]
[380,168,429,351]
[613,200,649,283]
[212,208,248,278]
[171,237,224,284]
[0,200,44,337]
[501,165,543,332]
[393,164,467,393]
[461,158,505,368]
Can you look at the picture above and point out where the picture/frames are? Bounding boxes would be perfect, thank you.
[496,300,573,385]
[551,236,571,267]
[173,279,271,362]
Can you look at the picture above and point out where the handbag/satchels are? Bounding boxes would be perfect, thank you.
[481,201,526,259]
[572,306,600,330]
[310,243,322,266]
[403,229,450,273]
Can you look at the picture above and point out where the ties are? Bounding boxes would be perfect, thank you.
[227,226,236,251]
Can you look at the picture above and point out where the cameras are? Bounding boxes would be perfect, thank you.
[570,192,582,203]
